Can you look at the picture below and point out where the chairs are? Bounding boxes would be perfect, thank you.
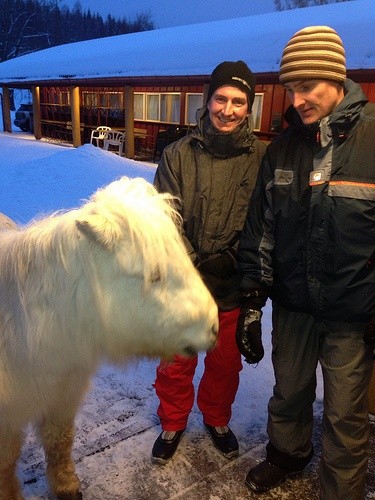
[90,126,125,156]
[55,121,85,141]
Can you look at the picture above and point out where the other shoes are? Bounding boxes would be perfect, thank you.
[151,429,185,465]
[203,420,239,459]
[244,442,316,493]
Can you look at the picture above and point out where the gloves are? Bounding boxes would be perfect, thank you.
[236,305,265,365]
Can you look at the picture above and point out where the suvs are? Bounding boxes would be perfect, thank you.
[14,103,33,132]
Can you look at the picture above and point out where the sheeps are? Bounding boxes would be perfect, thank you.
[0,176,219,500]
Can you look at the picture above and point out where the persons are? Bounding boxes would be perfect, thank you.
[150,61,269,467]
[236,25,375,499]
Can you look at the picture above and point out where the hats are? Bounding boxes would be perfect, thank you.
[278,25,348,85]
[206,61,257,113]
